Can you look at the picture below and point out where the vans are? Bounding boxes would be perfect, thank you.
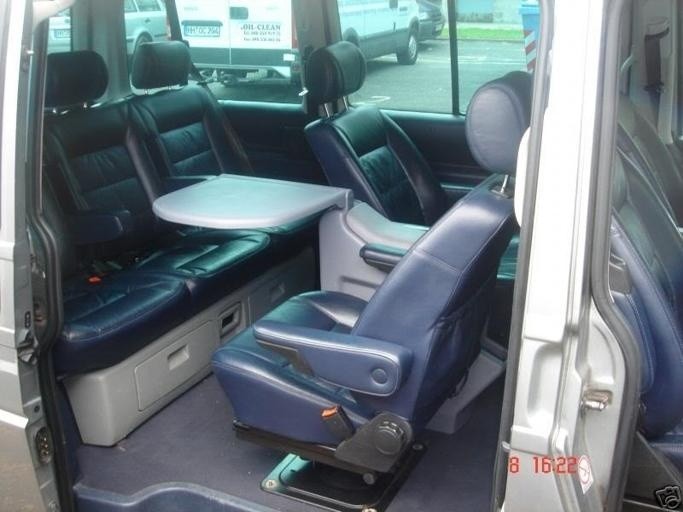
[169,2,421,90]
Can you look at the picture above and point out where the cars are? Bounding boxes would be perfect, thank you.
[417,2,446,39]
[46,1,169,57]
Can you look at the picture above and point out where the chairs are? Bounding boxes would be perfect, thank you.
[285,43,525,329]
[606,102,680,484]
[43,42,320,447]
[210,67,536,482]
